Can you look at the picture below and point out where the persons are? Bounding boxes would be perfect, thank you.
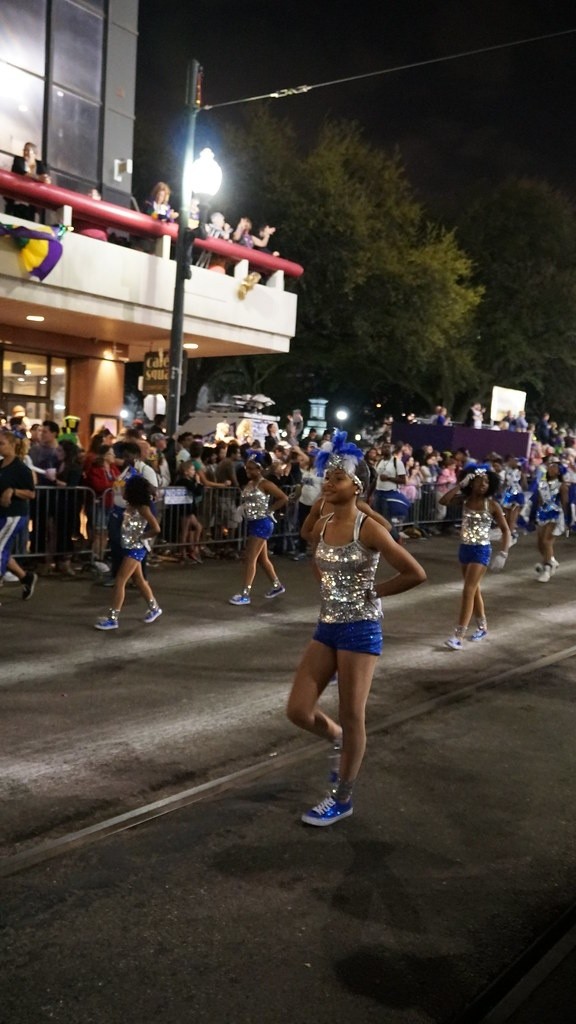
[142,181,280,288]
[284,431,428,826]
[0,400,576,649]
[4,142,53,223]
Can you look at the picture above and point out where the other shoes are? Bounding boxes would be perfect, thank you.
[4,571,19,582]
[151,554,163,563]
[471,626,488,641]
[59,561,76,576]
[553,560,559,575]
[20,572,38,600]
[191,553,204,564]
[396,523,458,539]
[104,578,120,588]
[536,564,552,582]
[201,548,216,557]
[293,552,306,560]
[445,636,464,649]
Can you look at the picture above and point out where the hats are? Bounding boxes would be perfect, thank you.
[458,463,500,498]
[151,433,170,443]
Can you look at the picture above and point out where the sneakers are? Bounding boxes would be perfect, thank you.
[229,594,251,604]
[327,736,342,783]
[301,792,354,826]
[144,607,162,623]
[265,585,285,598]
[94,619,119,630]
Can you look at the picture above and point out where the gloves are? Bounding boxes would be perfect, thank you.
[268,511,278,523]
[491,550,507,571]
[140,538,152,552]
[459,473,475,488]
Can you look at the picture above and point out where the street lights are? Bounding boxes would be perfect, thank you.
[165,58,224,449]
